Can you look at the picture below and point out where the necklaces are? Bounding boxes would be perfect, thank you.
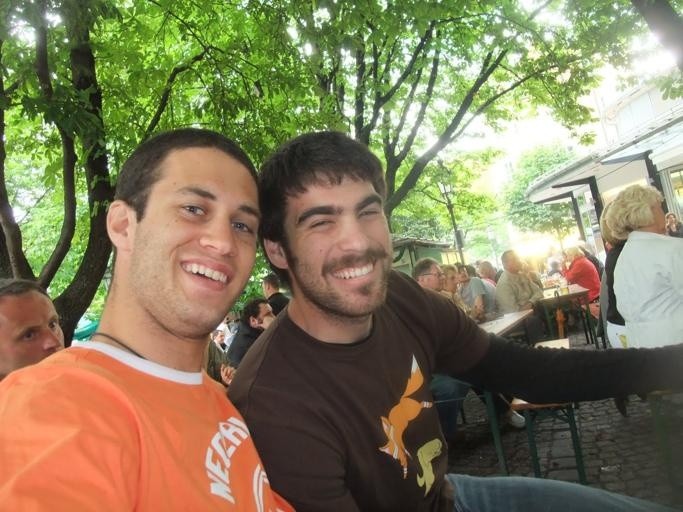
[88,330,147,360]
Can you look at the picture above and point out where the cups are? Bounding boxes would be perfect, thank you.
[560,279,569,292]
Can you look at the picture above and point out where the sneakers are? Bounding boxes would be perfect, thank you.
[508,411,525,429]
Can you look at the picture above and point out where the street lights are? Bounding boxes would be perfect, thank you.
[431,157,465,264]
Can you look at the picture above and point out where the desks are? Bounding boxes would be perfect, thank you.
[428,307,536,476]
[539,282,601,349]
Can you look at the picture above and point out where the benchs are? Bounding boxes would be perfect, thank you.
[509,338,590,484]
[580,300,609,351]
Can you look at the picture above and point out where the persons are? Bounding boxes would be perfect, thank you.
[2,275,65,380]
[228,134,683,512]
[1,130,296,512]
[412,240,606,345]
[206,266,291,386]
[593,184,683,349]
